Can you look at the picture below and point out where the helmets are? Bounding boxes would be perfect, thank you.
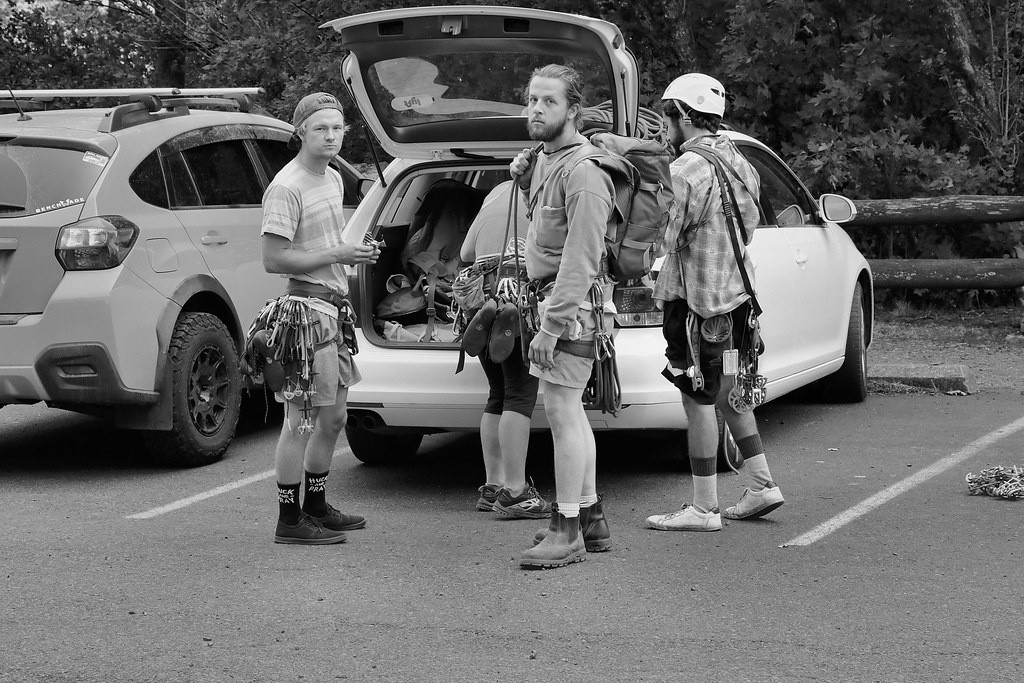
[661,73,726,120]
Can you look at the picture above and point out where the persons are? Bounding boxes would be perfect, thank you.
[645,72,785,531]
[260,92,381,545]
[510,64,619,568]
[460,178,554,519]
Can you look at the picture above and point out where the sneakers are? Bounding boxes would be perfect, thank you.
[644,501,722,532]
[724,481,784,519]
[313,503,366,530]
[493,475,552,518]
[476,484,497,511]
[274,510,347,545]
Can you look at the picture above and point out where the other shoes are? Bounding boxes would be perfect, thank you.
[489,303,518,363]
[463,299,498,357]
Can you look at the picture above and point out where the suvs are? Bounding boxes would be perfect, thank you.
[315,7,874,474]
[0,85,370,464]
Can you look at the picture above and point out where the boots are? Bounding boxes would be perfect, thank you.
[534,492,612,552]
[519,501,586,568]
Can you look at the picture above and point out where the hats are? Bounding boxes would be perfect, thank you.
[286,92,343,151]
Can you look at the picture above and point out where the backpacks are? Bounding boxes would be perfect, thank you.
[561,131,675,279]
